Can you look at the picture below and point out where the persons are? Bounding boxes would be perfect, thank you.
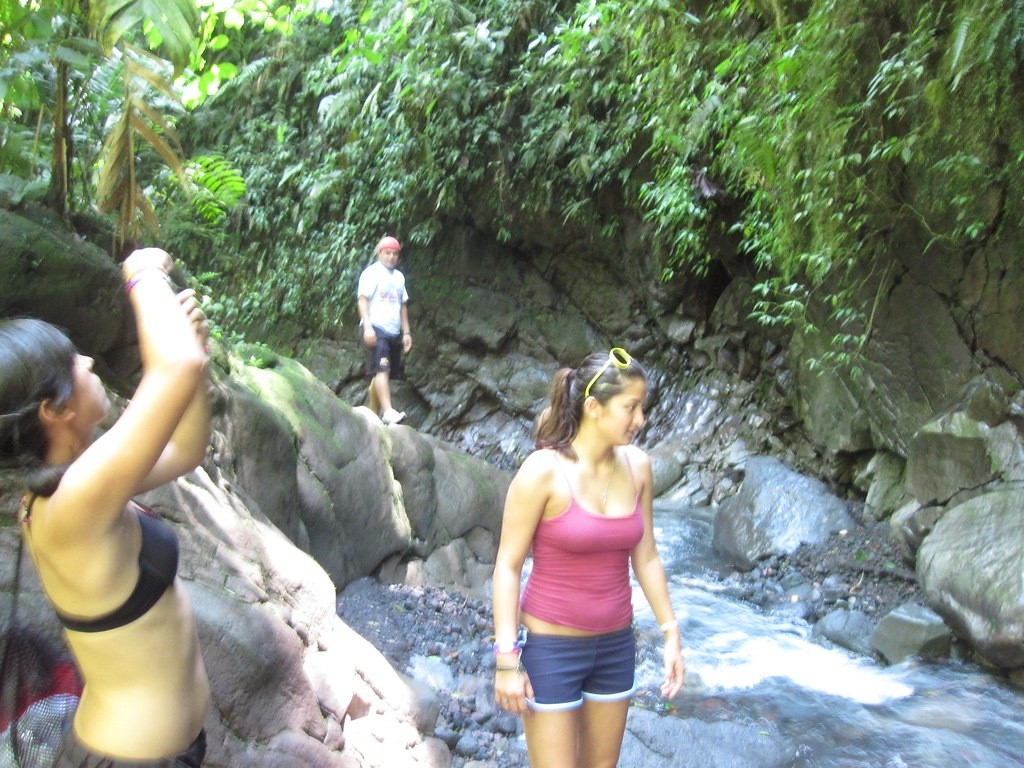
[353,236,414,427]
[489,345,684,768]
[2,244,217,768]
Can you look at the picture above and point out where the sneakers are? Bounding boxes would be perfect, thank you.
[383,409,406,423]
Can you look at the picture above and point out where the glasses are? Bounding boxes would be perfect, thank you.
[583,348,630,398]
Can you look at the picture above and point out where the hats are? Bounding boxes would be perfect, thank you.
[376,236,400,251]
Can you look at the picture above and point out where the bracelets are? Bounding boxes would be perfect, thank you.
[124,265,172,295]
[493,642,522,657]
[659,619,679,632]
[494,663,526,673]
[403,331,412,336]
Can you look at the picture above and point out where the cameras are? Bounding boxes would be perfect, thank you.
[163,270,187,292]
